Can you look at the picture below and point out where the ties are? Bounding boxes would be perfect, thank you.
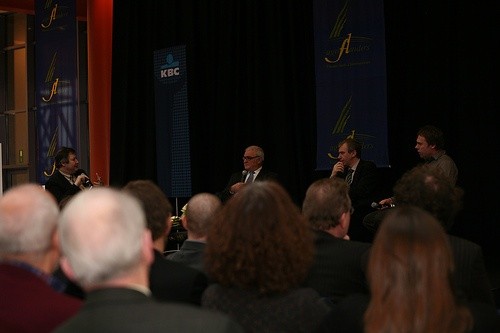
[345,169,354,184]
[246,171,255,184]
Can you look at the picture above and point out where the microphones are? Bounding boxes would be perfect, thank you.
[82,173,91,186]
[241,171,247,183]
[372,202,396,208]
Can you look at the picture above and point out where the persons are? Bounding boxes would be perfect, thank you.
[45,147,94,205]
[223,146,266,200]
[362,126,458,232]
[0,163,500,333]
[330,140,370,186]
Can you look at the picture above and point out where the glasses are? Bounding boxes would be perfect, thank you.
[242,156,260,161]
